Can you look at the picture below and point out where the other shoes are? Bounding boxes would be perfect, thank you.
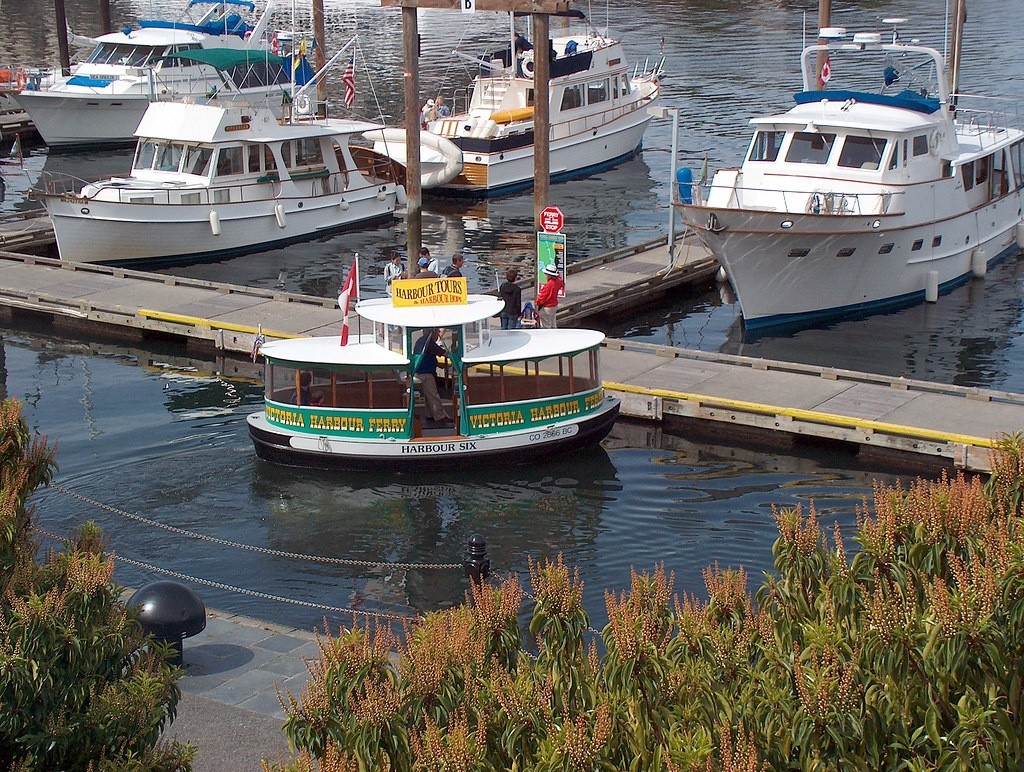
[436,417,453,424]
[425,416,436,423]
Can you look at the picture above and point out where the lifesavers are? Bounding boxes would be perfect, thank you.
[17,68,27,90]
[295,94,311,114]
[930,130,940,157]
[522,57,534,78]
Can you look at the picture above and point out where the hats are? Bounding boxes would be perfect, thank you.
[542,264,560,276]
[417,258,430,267]
[426,98,435,106]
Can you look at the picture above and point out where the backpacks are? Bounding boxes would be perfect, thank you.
[439,266,458,278]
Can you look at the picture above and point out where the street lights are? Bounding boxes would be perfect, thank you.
[646,106,680,267]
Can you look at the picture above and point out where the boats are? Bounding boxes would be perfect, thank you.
[670,10,1024,329]
[246,277,620,472]
[18,0,319,152]
[418,0,668,200]
[361,127,464,191]
[20,34,411,268]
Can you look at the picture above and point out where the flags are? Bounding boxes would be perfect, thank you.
[272,32,319,70]
[337,257,357,346]
[341,50,355,110]
[698,157,708,185]
[818,57,832,85]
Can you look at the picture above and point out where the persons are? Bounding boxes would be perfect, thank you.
[414,328,454,423]
[291,372,324,405]
[420,95,450,130]
[508,32,533,59]
[536,265,563,328]
[383,247,464,343]
[500,269,522,330]
